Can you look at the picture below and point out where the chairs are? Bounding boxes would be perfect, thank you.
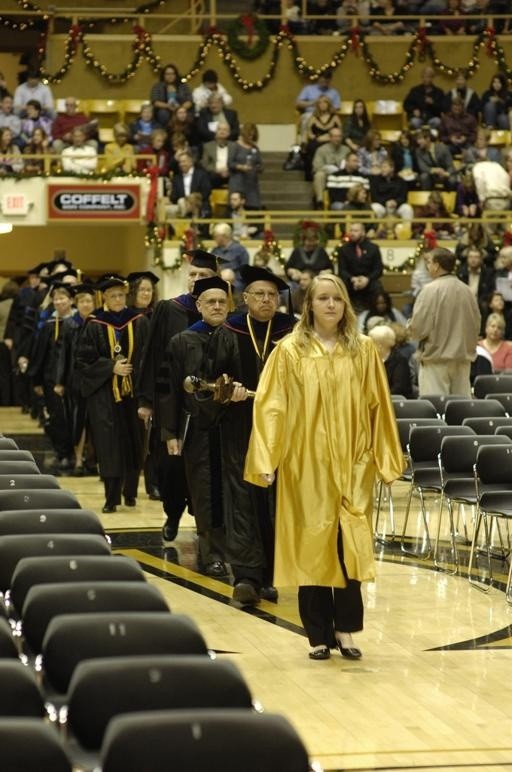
[1,658,49,722]
[1,619,22,658]
[1,437,20,449]
[10,554,146,613]
[18,90,255,216]
[1,449,36,461]
[40,610,209,690]
[20,580,171,652]
[95,705,309,770]
[321,90,512,213]
[1,473,60,489]
[384,375,512,594]
[1,487,81,511]
[0,535,112,590]
[68,653,256,750]
[1,509,104,540]
[1,460,40,474]
[1,711,71,772]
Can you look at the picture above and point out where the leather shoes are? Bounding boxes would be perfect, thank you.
[201,560,228,577]
[163,516,180,541]
[102,489,161,513]
[309,628,362,660]
[233,582,278,604]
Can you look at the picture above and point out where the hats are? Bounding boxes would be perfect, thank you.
[184,248,295,328]
[28,258,160,298]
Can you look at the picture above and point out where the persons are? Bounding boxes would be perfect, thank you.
[1,222,512,606]
[1,60,263,240]
[253,0,512,36]
[284,68,512,239]
[240,270,405,662]
